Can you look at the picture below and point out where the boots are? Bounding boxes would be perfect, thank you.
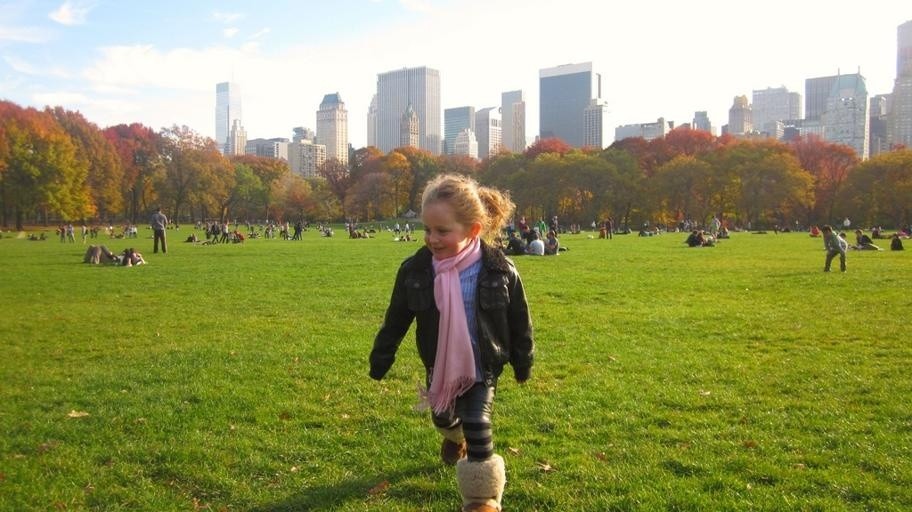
[456,454,506,512]
[436,420,466,464]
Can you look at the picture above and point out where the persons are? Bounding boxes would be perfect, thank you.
[344,221,418,242]
[26,220,146,267]
[150,208,168,253]
[638,216,729,248]
[368,173,534,512]
[773,216,912,251]
[734,220,752,232]
[591,216,632,239]
[822,224,848,273]
[496,215,580,256]
[167,214,333,246]
